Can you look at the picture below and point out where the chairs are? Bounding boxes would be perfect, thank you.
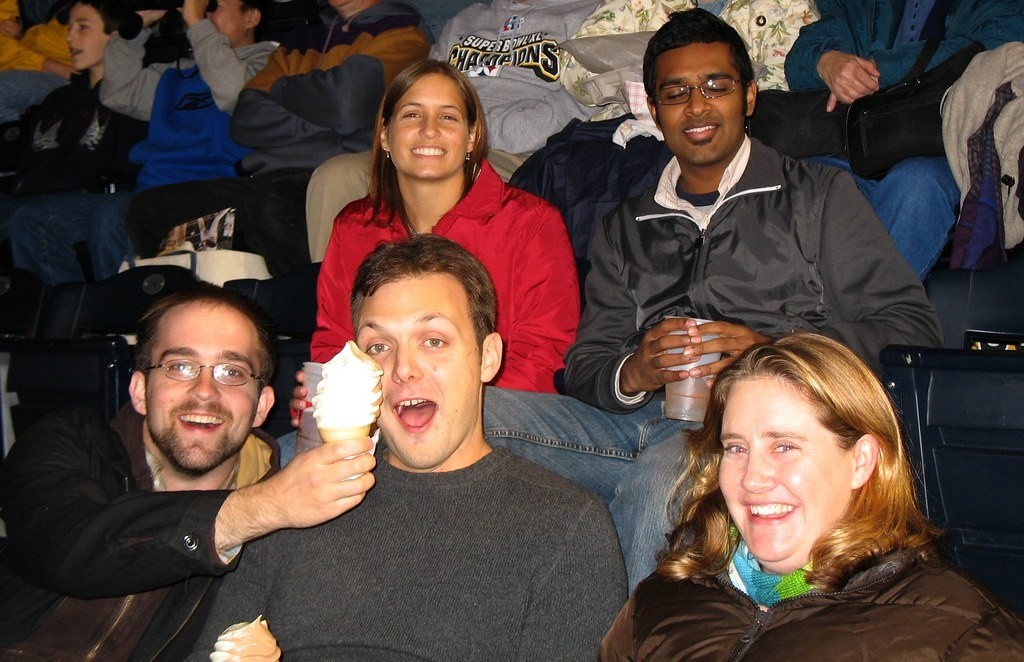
[877,341,1024,623]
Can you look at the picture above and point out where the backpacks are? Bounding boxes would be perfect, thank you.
[510,110,644,314]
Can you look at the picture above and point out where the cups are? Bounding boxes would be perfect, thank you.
[297,362,325,454]
[665,316,725,423]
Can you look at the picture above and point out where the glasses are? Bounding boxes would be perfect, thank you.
[652,77,750,106]
[133,358,268,386]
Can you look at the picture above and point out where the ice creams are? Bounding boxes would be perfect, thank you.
[312,339,384,444]
[209,614,281,662]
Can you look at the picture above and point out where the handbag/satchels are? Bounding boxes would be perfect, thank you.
[843,33,989,181]
[745,89,849,159]
[55,245,267,436]
[144,205,237,259]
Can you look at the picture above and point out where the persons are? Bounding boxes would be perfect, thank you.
[274,58,581,467]
[186,235,631,662]
[304,1,603,263]
[484,7,942,595]
[597,332,1024,662]
[558,0,822,138]
[934,260,1024,352]
[0,286,375,662]
[784,0,1024,287]
[0,0,436,285]
